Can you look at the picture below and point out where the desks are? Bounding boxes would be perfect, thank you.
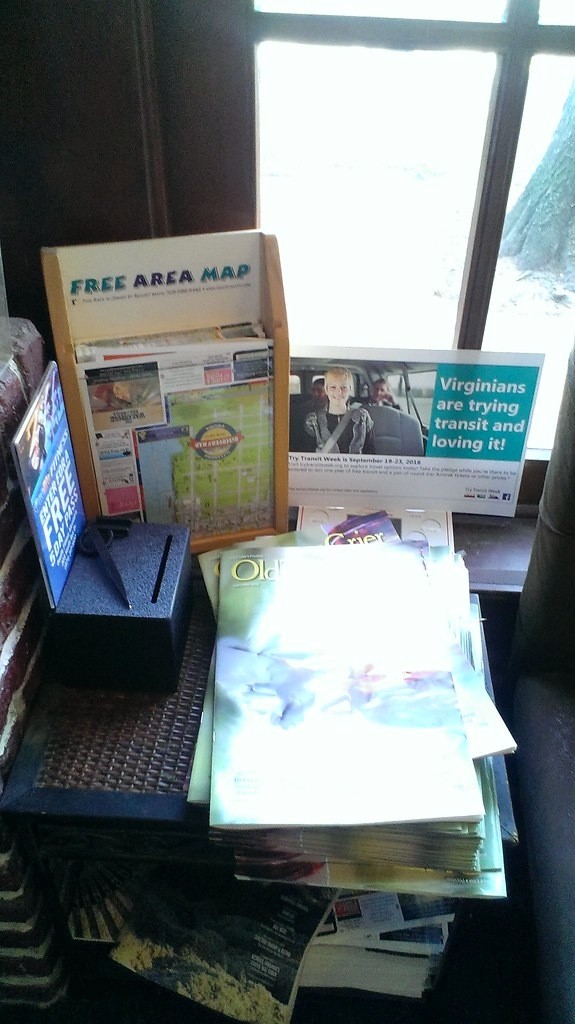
[0,587,519,1024]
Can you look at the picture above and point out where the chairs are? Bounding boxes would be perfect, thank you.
[369,405,424,456]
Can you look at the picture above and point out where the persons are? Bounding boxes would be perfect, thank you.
[294,366,402,455]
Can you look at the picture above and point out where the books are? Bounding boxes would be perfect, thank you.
[68,511,521,1024]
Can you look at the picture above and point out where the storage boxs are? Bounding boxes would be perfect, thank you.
[51,522,192,692]
